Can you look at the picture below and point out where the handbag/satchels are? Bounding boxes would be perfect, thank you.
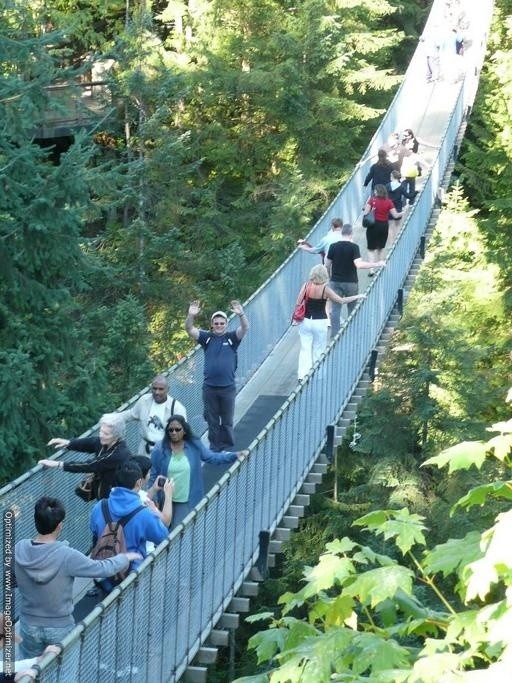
[76,474,93,502]
[363,213,375,226]
[293,304,305,320]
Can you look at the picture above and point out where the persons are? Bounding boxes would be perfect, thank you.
[182,297,251,450]
[419,0,470,84]
[39,376,252,594]
[3,497,141,665]
[295,126,423,387]
[0,608,65,683]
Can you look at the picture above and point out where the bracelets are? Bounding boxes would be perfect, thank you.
[239,311,245,317]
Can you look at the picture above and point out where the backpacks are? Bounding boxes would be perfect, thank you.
[91,499,145,581]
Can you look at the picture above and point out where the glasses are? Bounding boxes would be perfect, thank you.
[169,428,181,432]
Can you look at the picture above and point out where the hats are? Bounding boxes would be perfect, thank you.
[212,311,227,319]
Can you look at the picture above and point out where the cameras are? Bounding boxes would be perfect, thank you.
[156,478,172,487]
